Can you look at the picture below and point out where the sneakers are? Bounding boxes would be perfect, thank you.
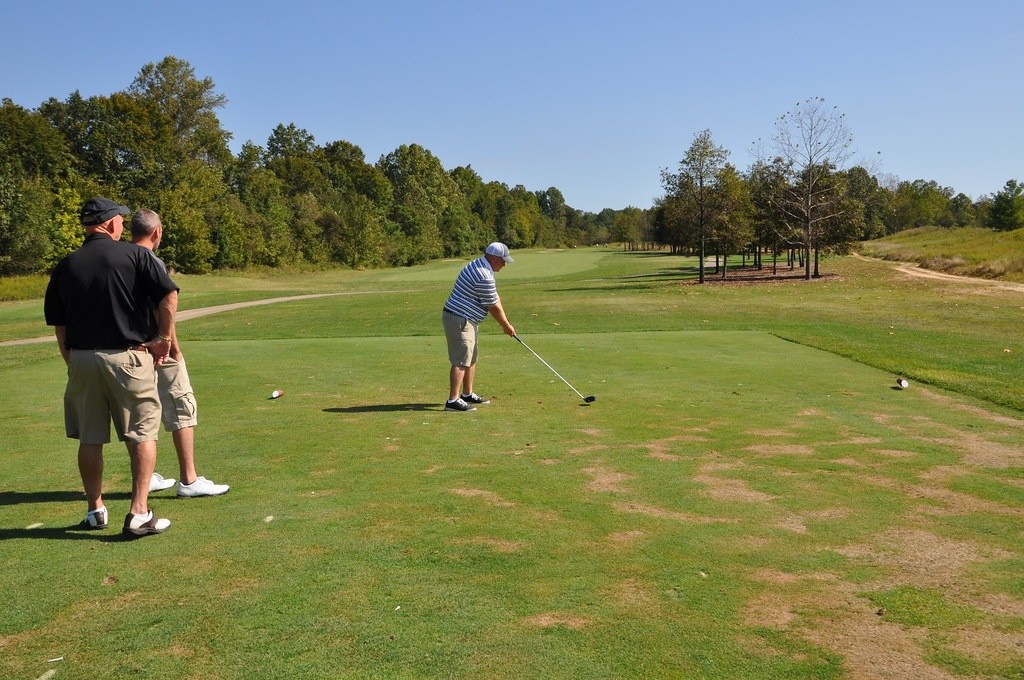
[148,474,176,493]
[177,476,231,498]
[79,509,108,528]
[445,398,476,411]
[123,508,170,538]
[460,393,490,404]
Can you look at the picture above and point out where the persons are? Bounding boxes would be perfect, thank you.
[442,242,516,412]
[43,198,181,535]
[129,207,229,498]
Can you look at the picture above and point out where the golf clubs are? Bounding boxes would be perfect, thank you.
[513,334,597,404]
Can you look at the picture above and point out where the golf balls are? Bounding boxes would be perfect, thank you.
[272,390,279,398]
[901,380,908,388]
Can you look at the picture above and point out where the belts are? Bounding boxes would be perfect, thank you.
[443,307,454,313]
[119,346,147,351]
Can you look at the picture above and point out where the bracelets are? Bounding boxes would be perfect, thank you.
[158,334,173,342]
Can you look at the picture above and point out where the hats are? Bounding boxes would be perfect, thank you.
[485,242,514,263]
[80,198,129,226]
